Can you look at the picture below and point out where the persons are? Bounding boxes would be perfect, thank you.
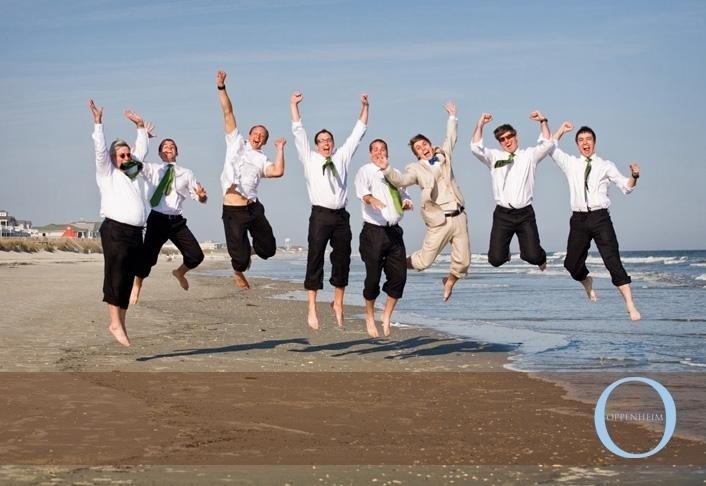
[290,88,371,331]
[547,121,643,322]
[370,99,472,302]
[128,120,208,306]
[89,98,151,349]
[214,69,288,293]
[470,110,554,272]
[351,138,415,338]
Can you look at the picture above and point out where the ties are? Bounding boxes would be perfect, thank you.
[384,176,403,215]
[323,157,336,177]
[495,152,514,168]
[584,159,591,190]
[120,160,143,178]
[149,164,173,207]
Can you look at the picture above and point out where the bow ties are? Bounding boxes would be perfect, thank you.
[428,156,439,164]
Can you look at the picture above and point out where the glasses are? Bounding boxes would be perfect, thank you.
[119,153,131,159]
[319,138,333,143]
[498,133,515,142]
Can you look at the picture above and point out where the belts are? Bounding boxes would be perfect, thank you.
[153,211,181,220]
[444,206,464,217]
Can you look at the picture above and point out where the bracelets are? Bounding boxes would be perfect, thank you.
[539,118,548,123]
[631,172,640,179]
[217,84,226,90]
